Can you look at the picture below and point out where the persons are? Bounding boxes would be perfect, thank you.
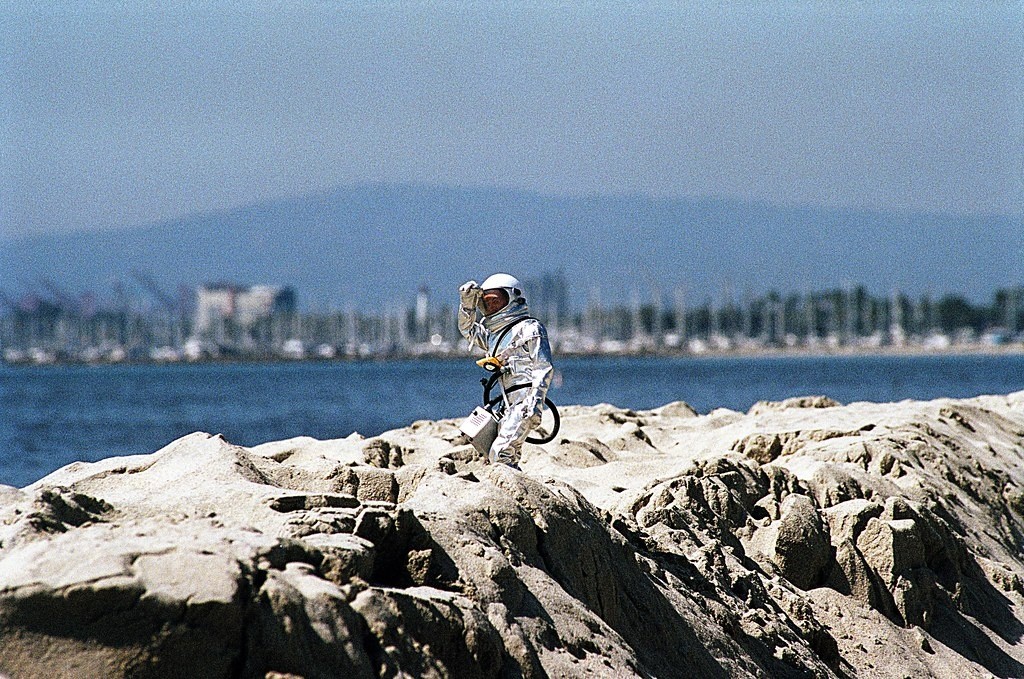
[457,269,560,473]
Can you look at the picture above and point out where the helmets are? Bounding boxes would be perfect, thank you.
[477,271,523,315]
